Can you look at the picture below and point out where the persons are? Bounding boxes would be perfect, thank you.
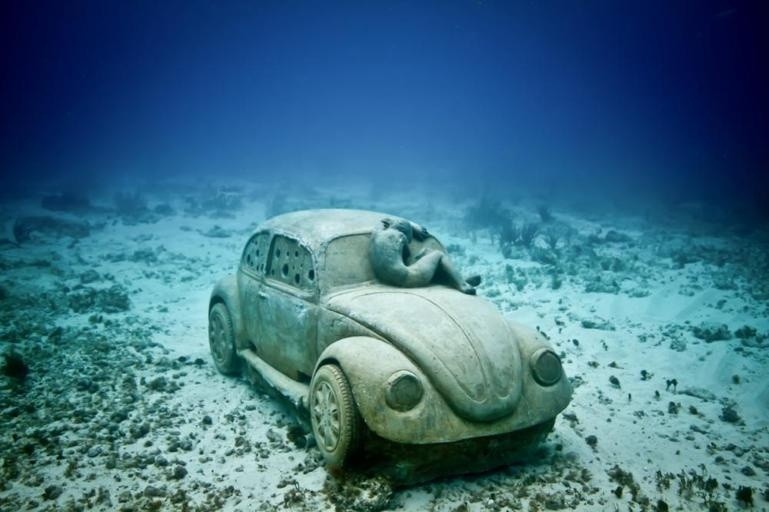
[369,218,481,295]
